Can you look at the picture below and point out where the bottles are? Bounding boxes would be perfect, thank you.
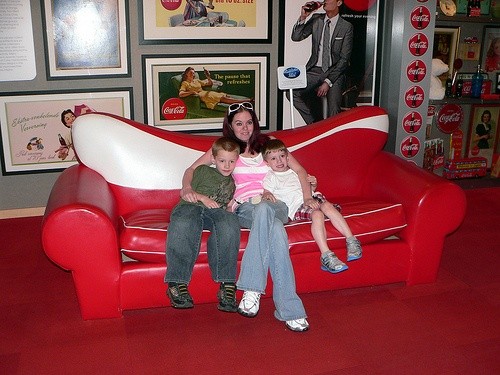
[202,67,210,77]
[304,1,325,12]
[414,61,419,81]
[412,87,417,107]
[408,137,413,158]
[58,133,68,149]
[409,113,415,132]
[422,140,441,167]
[415,33,421,55]
[417,6,423,29]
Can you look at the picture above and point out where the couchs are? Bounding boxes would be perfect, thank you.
[40,105,468,321]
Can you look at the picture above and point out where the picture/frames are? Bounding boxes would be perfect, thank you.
[0,0,274,178]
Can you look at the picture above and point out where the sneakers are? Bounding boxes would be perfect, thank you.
[166,283,193,308]
[239,290,261,316]
[321,252,348,274]
[285,317,310,331]
[346,240,362,261]
[217,282,238,312]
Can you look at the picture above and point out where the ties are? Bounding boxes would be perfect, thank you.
[322,19,331,73]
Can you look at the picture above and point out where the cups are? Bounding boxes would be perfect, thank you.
[470,64,484,97]
[455,75,464,98]
[195,3,222,27]
[445,74,451,97]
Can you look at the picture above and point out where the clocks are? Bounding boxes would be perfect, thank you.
[439,0,457,16]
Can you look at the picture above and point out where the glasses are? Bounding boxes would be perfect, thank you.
[228,102,253,115]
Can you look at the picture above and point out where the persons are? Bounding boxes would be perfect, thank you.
[286,0,353,125]
[164,139,240,312]
[178,67,253,109]
[182,101,319,332]
[473,110,494,159]
[261,139,362,273]
